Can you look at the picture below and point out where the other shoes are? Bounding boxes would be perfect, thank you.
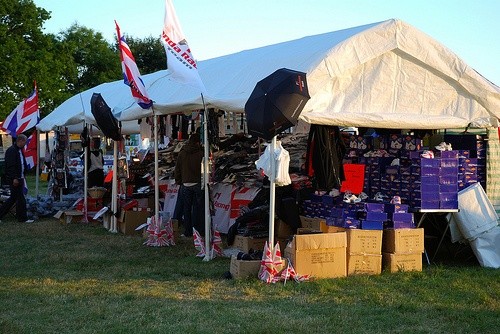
[196,251,204,257]
[203,255,215,261]
[25,219,35,222]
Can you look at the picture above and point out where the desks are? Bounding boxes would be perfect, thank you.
[163,181,260,235]
[410,207,460,266]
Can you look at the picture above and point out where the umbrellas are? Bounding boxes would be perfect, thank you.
[244,68,311,141]
[90,92,123,142]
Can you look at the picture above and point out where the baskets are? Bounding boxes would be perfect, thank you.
[88,187,107,198]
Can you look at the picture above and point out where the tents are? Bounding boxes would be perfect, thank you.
[35,18,500,282]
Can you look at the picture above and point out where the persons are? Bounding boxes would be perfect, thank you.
[173,126,205,238]
[80,137,105,196]
[0,134,34,222]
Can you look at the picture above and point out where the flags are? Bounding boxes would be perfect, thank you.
[144,216,175,246]
[0,86,39,195]
[113,19,155,110]
[193,228,224,260]
[162,0,205,90]
[258,241,309,286]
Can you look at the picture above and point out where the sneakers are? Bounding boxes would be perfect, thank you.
[345,139,452,166]
[314,188,402,204]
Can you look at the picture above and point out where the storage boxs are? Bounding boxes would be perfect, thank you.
[230,133,487,279]
[53,194,179,234]
